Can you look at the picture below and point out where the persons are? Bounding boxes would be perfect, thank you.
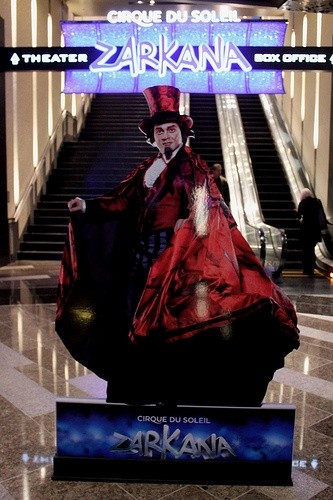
[67,106,244,414]
[208,162,230,211]
[296,188,329,278]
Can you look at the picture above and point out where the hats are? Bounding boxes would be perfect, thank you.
[138,86,193,134]
